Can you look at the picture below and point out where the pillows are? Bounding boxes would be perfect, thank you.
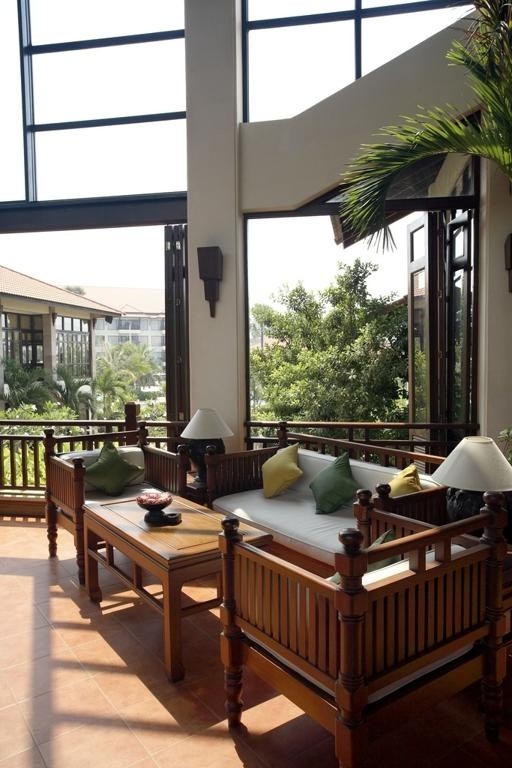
[306,449,365,518]
[78,436,148,498]
[381,457,428,501]
[328,525,408,587]
[256,439,304,501]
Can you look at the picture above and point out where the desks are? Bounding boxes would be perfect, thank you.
[183,470,205,500]
[68,493,276,682]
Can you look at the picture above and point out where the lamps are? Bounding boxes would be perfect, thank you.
[179,406,236,481]
[195,243,226,318]
[431,432,511,535]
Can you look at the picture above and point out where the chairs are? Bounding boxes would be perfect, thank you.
[39,418,188,587]
[211,489,510,765]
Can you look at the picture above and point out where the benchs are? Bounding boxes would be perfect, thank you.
[202,443,445,569]
